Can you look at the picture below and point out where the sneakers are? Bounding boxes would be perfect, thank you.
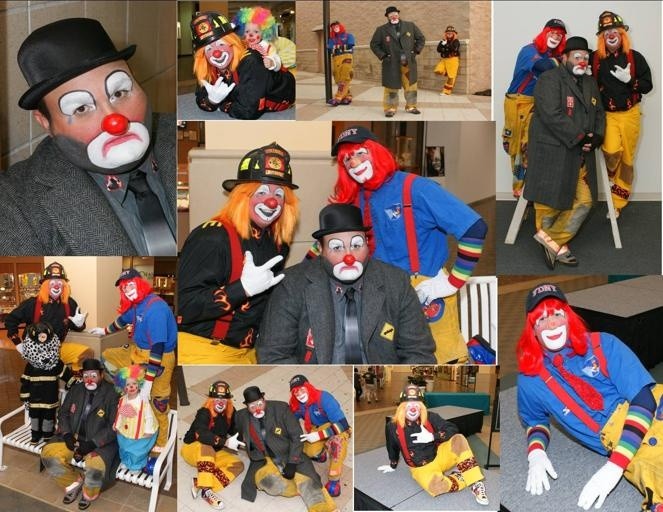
[471,482,490,505]
[325,480,340,497]
[192,477,200,498]
[30,437,38,445]
[440,92,449,96]
[78,494,90,510]
[559,248,578,266]
[468,334,496,362]
[385,111,394,117]
[412,109,420,114]
[63,474,84,504]
[327,98,338,107]
[606,208,620,219]
[451,470,463,479]
[316,449,327,463]
[201,489,224,509]
[342,95,352,105]
[541,242,555,269]
[146,456,160,473]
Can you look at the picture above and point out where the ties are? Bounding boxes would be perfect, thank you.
[576,76,583,92]
[344,287,363,365]
[258,418,275,457]
[77,390,95,442]
[126,169,177,256]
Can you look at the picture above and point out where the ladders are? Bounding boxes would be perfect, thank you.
[504,144,624,249]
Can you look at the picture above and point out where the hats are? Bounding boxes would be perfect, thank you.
[205,381,234,399]
[596,11,629,35]
[39,262,70,285]
[79,358,105,371]
[290,375,308,391]
[242,386,265,404]
[330,18,336,26]
[222,142,299,191]
[18,18,137,110]
[545,18,567,34]
[525,283,568,313]
[561,36,592,53]
[399,384,424,402]
[115,269,141,287]
[330,125,377,156]
[445,26,455,32]
[191,10,239,52]
[385,7,400,17]
[311,202,373,239]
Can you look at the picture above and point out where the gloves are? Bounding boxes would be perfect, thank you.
[88,327,105,336]
[282,463,296,479]
[299,418,308,434]
[415,276,458,306]
[410,423,434,443]
[577,460,623,511]
[63,433,76,451]
[377,465,395,473]
[525,449,558,496]
[227,432,245,451]
[240,250,285,297]
[440,39,447,46]
[67,306,88,328]
[140,380,153,402]
[15,343,23,354]
[578,130,591,146]
[76,439,96,456]
[590,132,602,151]
[200,77,236,104]
[609,63,631,84]
[299,431,320,444]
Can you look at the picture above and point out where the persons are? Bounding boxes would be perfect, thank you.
[329,21,355,107]
[302,127,487,364]
[0,18,177,256]
[178,143,301,363]
[235,386,330,508]
[289,375,352,497]
[589,11,653,221]
[42,357,120,508]
[113,367,159,474]
[190,12,293,119]
[378,384,490,505]
[434,25,460,96]
[503,19,567,199]
[89,269,178,476]
[369,6,426,118]
[518,283,663,508]
[256,203,437,363]
[6,263,87,446]
[232,8,286,71]
[181,382,244,508]
[523,36,608,271]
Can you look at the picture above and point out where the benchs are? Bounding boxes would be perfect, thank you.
[0,388,177,512]
